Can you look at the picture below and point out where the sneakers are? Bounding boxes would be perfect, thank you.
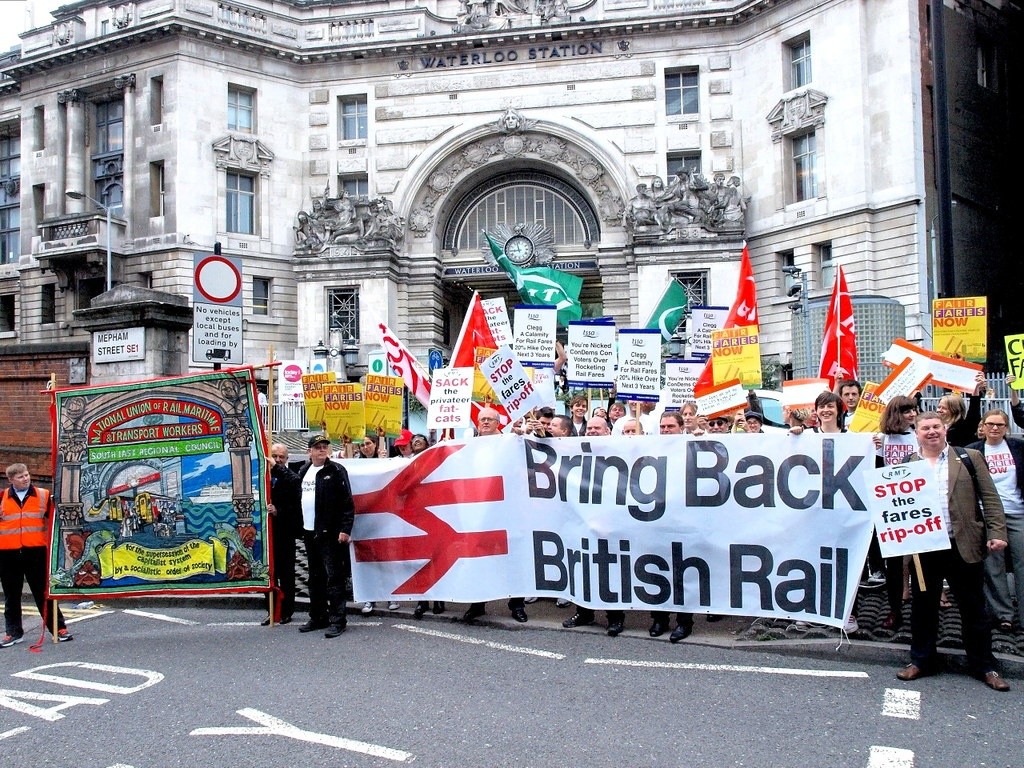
[843,615,858,634]
[0,634,24,647]
[388,601,400,609]
[298,620,330,632]
[524,597,570,608]
[362,601,375,613]
[52,628,73,642]
[325,624,347,637]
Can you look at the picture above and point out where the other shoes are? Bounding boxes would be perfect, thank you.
[882,612,903,629]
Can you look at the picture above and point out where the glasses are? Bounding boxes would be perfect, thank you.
[477,417,498,423]
[708,420,723,427]
[315,445,329,450]
[983,422,1007,429]
[411,437,424,442]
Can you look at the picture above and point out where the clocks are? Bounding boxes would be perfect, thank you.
[504,234,535,265]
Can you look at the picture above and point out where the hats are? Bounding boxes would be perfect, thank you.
[394,429,413,447]
[308,435,331,448]
[744,411,763,423]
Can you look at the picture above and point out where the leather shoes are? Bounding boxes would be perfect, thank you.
[464,606,486,620]
[898,664,920,679]
[563,612,595,628]
[983,671,1011,690]
[670,623,693,642]
[607,618,625,636]
[649,619,670,637]
[512,608,529,622]
[261,614,270,625]
[415,601,445,616]
[280,617,292,624]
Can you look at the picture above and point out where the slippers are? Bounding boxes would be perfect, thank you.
[867,570,954,610]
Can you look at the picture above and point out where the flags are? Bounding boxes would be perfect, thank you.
[641,279,686,346]
[483,230,583,326]
[692,241,758,392]
[377,323,432,411]
[450,291,511,433]
[817,265,858,411]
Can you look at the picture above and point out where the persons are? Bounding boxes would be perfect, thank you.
[977,418,986,439]
[961,408,1024,632]
[937,372,985,446]
[261,444,298,625]
[267,436,354,638]
[0,463,73,650]
[898,411,1010,692]
[1005,374,1024,428]
[333,381,920,646]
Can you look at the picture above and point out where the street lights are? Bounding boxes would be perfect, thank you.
[931,199,958,299]
[66,189,111,292]
